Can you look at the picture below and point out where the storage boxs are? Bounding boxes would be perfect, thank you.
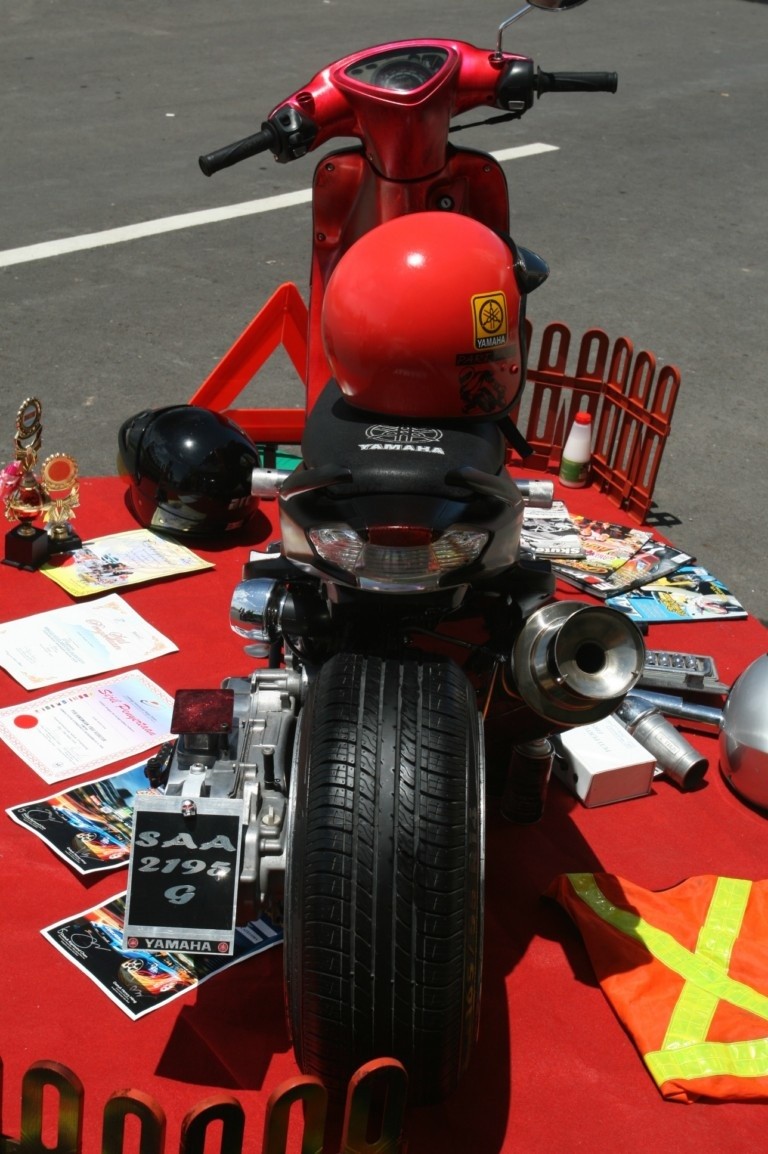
[552,714,657,807]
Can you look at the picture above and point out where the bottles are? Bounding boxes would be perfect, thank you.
[500,737,556,826]
[617,696,710,789]
[559,412,592,488]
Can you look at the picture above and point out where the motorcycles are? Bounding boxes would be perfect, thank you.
[120,0,652,1122]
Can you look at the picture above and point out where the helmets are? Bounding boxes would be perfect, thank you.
[318,209,550,422]
[115,403,265,535]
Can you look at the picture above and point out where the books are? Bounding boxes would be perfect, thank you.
[518,500,749,624]
